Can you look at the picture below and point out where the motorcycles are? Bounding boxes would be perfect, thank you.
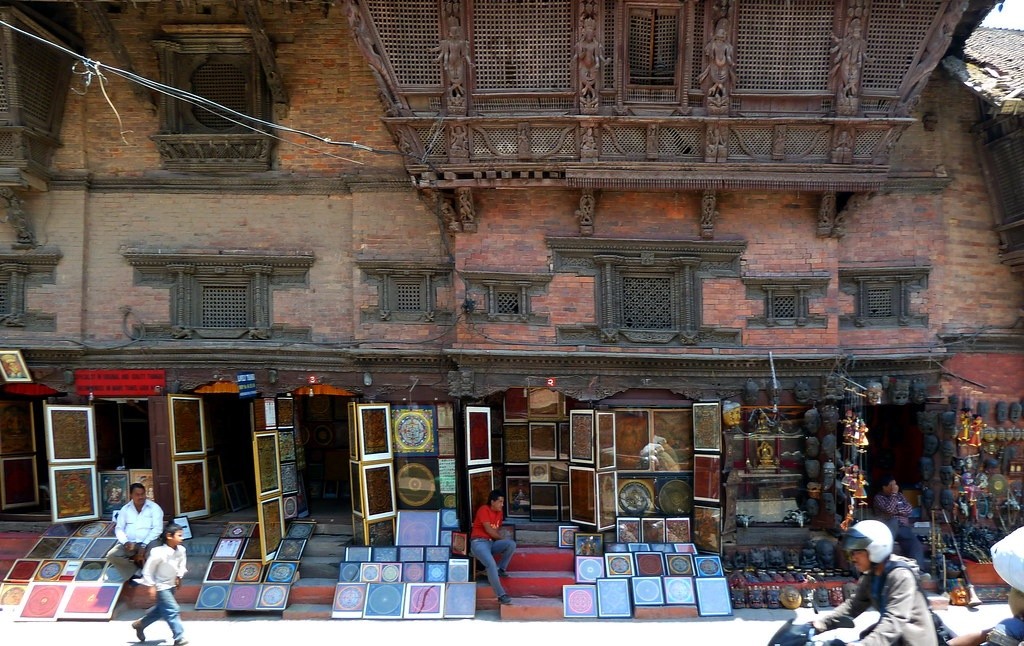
[767,600,959,646]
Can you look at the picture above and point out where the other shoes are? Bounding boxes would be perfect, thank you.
[132,619,145,642]
[174,639,189,646]
[498,594,512,604]
[498,568,511,578]
[919,570,931,582]
[128,573,145,587]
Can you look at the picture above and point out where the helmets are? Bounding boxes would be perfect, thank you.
[842,519,894,564]
[991,526,1024,593]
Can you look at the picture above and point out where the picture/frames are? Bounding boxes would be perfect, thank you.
[0,348,733,624]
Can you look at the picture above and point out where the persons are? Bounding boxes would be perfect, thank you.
[574,18,608,97]
[829,19,873,98]
[945,588,1024,646]
[470,490,517,605]
[105,483,164,588]
[131,523,190,646]
[873,477,932,581]
[426,17,476,98]
[705,26,737,99]
[806,520,939,646]
